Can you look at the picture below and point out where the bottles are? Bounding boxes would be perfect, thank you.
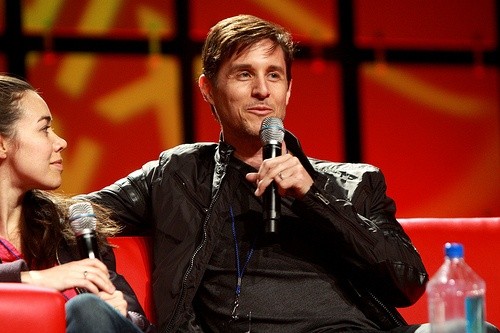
[425,242,487,333]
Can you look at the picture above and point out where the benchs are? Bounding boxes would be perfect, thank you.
[0,218,500,333]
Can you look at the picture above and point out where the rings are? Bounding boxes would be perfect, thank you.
[84,271,87,277]
[278,173,285,180]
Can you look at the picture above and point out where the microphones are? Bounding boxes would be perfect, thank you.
[68,201,101,259]
[259,116,285,231]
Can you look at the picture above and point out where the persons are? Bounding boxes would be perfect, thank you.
[74,13,430,333]
[0,76,143,333]
